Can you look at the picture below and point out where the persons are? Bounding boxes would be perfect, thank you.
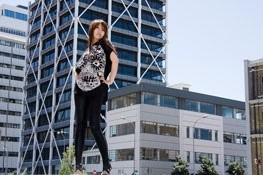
[131,169,138,175]
[72,19,119,175]
[91,169,96,175]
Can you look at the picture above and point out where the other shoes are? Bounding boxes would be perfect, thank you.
[73,169,83,175]
[101,165,112,175]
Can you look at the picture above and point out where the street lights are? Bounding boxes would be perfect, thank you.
[120,116,136,175]
[192,115,208,175]
[3,148,9,175]
[57,132,65,155]
